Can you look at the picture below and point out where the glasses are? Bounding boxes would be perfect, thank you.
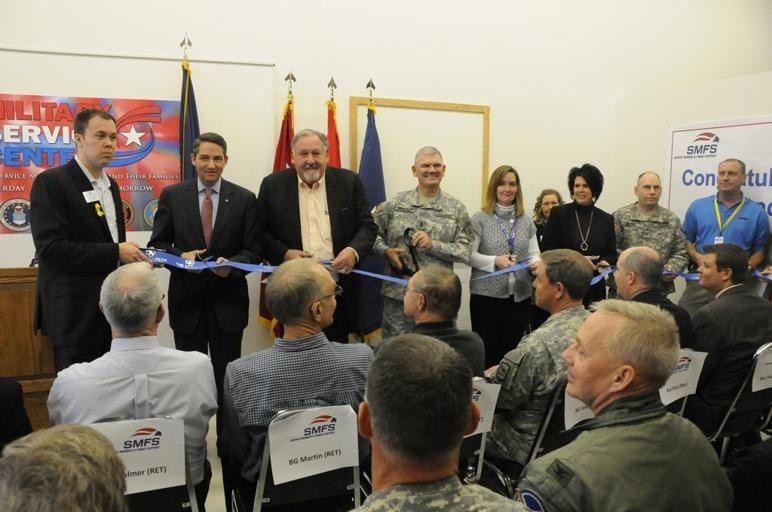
[311,284,344,312]
[402,283,422,295]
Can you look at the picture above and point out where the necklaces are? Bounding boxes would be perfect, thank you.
[574,207,596,251]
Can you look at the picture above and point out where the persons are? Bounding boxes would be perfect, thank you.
[727,436,772,512]
[220,258,376,511]
[0,377,33,460]
[360,265,485,489]
[30,109,154,378]
[530,189,563,252]
[0,424,129,512]
[605,172,689,300]
[46,262,219,512]
[469,165,541,371]
[677,159,770,319]
[258,128,379,344]
[485,249,594,480]
[613,245,694,415]
[541,163,619,310]
[146,132,264,458]
[511,300,735,512]
[371,146,475,340]
[683,243,771,441]
[345,333,533,512]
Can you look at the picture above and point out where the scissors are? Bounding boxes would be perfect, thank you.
[593,255,610,275]
[391,226,421,277]
[196,249,217,274]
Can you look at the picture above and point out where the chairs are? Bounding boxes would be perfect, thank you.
[683,341,771,467]
[87,416,199,512]
[474,347,709,498]
[230,404,360,511]
[462,377,502,486]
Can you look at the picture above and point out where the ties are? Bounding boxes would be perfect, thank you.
[201,186,213,250]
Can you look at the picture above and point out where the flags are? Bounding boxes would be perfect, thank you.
[358,77,387,212]
[324,76,341,168]
[178,32,200,184]
[273,71,297,175]
